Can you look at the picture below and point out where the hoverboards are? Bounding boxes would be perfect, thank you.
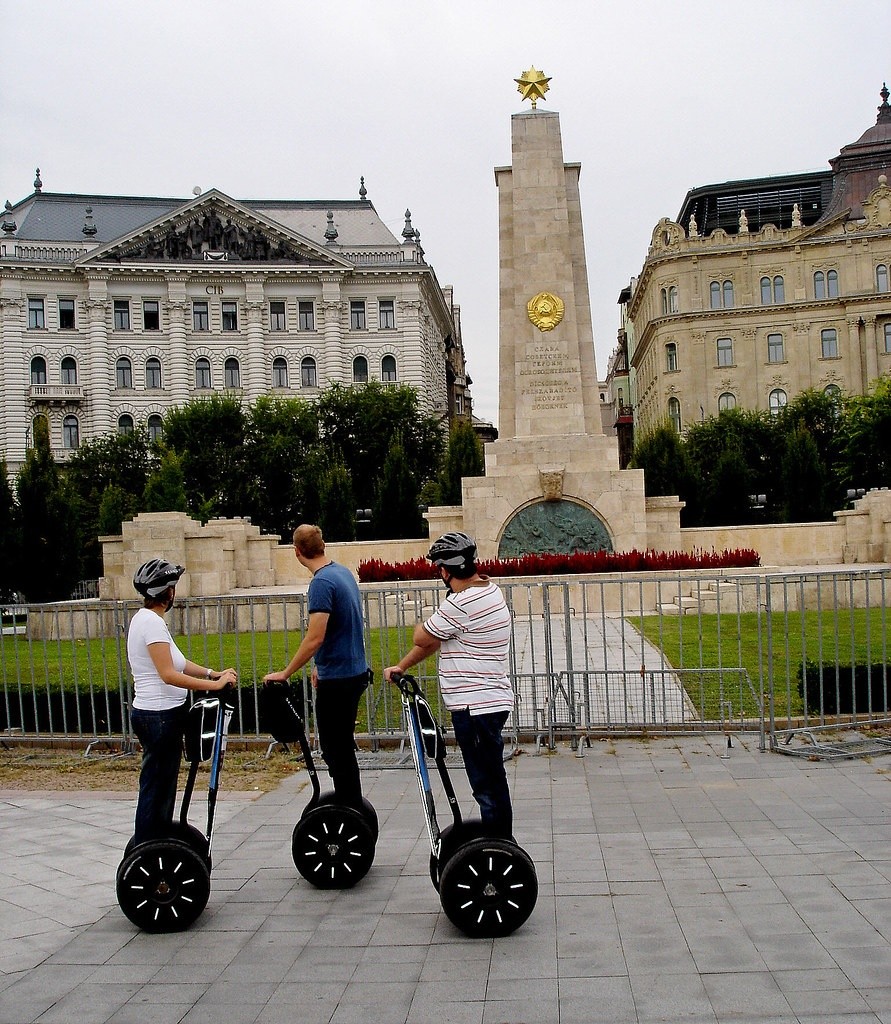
[118,677,232,933]
[264,681,378,890]
[392,672,538,939]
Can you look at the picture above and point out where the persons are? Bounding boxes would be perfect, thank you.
[124,559,238,843]
[383,533,515,843]
[262,524,370,820]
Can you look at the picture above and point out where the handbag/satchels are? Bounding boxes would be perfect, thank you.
[397,674,447,759]
[258,680,312,752]
[182,698,220,762]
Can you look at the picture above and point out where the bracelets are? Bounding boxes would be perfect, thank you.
[207,669,213,676]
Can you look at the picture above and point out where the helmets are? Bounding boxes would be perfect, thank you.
[133,558,186,598]
[426,531,479,570]
